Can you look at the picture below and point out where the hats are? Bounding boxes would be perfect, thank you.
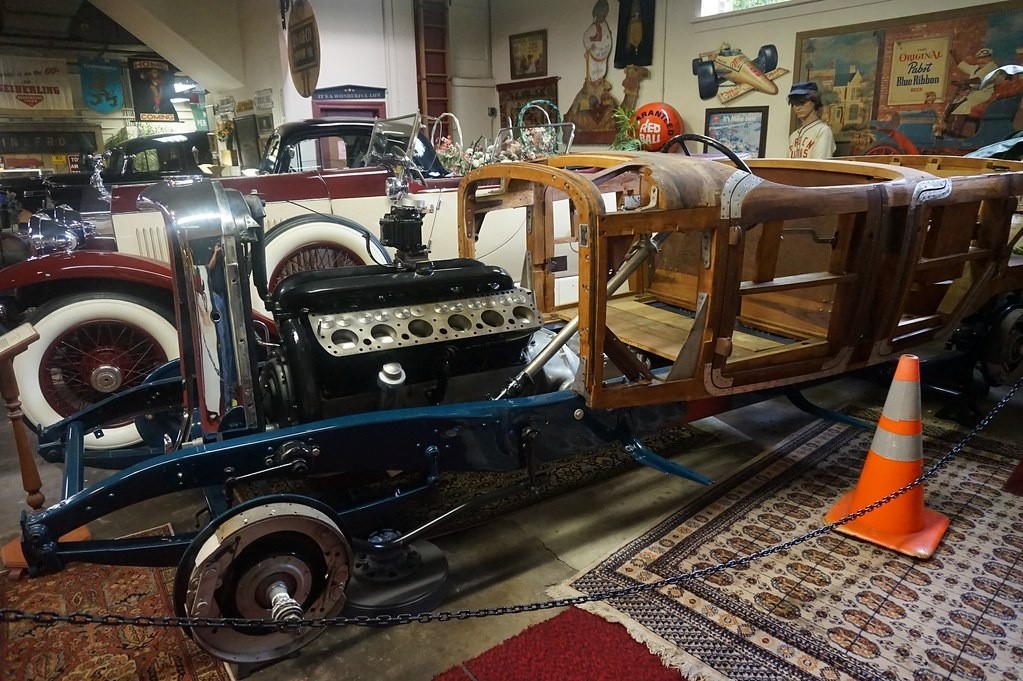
[787,82,818,96]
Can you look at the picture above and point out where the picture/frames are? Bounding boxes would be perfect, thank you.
[703,105,769,158]
[255,113,274,135]
[508,29,548,80]
[788,0,1023,141]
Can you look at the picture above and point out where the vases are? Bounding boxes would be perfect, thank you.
[226,137,234,149]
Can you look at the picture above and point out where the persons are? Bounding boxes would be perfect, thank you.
[787,82,837,158]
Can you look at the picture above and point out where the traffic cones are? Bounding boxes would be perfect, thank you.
[823,354,950,562]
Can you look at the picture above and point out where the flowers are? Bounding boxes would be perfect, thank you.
[215,115,234,142]
[432,126,557,174]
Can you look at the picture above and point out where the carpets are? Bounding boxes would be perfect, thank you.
[426,607,682,681]
[0,523,236,681]
[413,415,721,540]
[546,399,1023,681]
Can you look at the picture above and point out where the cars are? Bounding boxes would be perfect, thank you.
[0,103,1023,667]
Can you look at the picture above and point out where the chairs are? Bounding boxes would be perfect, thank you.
[349,136,365,168]
